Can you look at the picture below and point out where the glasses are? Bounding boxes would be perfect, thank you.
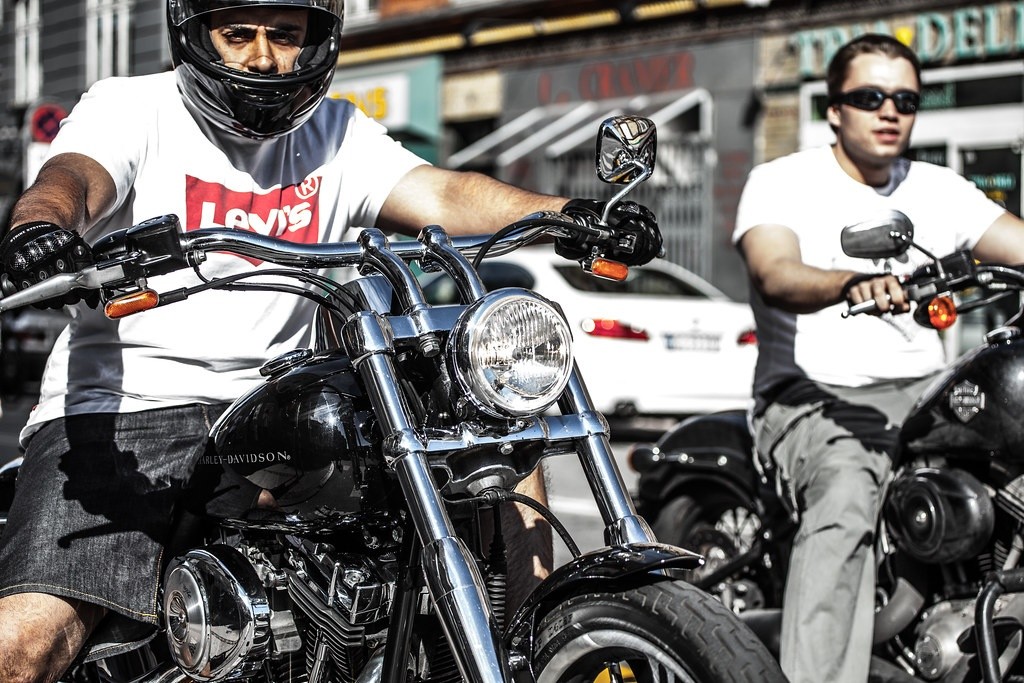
[831,87,921,115]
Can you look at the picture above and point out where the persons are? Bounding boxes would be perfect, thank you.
[0,0,662,683]
[733,34,1024,683]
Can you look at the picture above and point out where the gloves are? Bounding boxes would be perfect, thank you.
[0,222,103,309]
[557,199,663,265]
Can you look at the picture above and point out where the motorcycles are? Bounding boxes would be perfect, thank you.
[1,114,787,683]
[628,209,1024,683]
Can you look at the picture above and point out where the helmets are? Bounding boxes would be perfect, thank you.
[165,0,347,140]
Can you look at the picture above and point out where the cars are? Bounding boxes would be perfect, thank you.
[412,238,759,440]
